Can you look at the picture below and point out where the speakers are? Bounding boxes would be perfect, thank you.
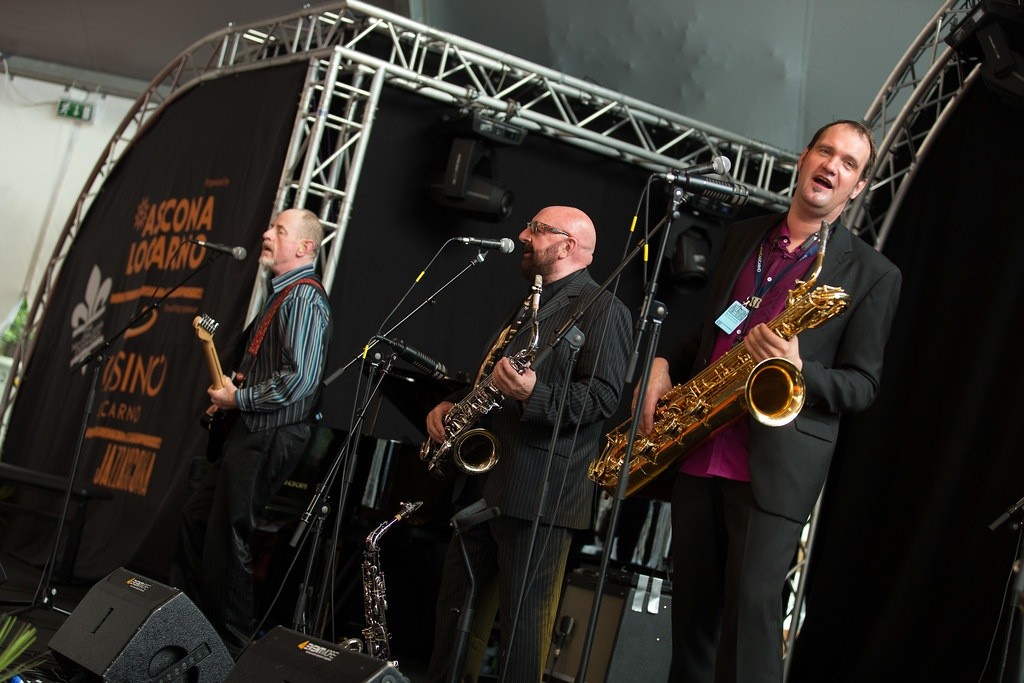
[542,572,674,683]
[224,625,409,683]
[48,566,235,683]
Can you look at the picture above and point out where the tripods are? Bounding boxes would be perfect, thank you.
[0,251,223,619]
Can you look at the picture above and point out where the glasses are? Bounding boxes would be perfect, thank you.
[527,221,571,237]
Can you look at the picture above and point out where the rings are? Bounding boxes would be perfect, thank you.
[631,405,635,408]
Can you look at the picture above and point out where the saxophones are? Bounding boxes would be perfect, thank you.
[587,220,853,501]
[330,500,423,660]
[413,274,548,482]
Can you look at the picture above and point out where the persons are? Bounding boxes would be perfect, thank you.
[426,205,633,683]
[129,209,335,660]
[628,118,902,683]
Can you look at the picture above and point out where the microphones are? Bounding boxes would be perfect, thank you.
[553,615,574,660]
[375,336,448,381]
[652,157,731,181]
[192,239,247,261]
[653,172,750,207]
[455,236,515,255]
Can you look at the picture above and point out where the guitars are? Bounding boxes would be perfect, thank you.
[193,315,248,466]
